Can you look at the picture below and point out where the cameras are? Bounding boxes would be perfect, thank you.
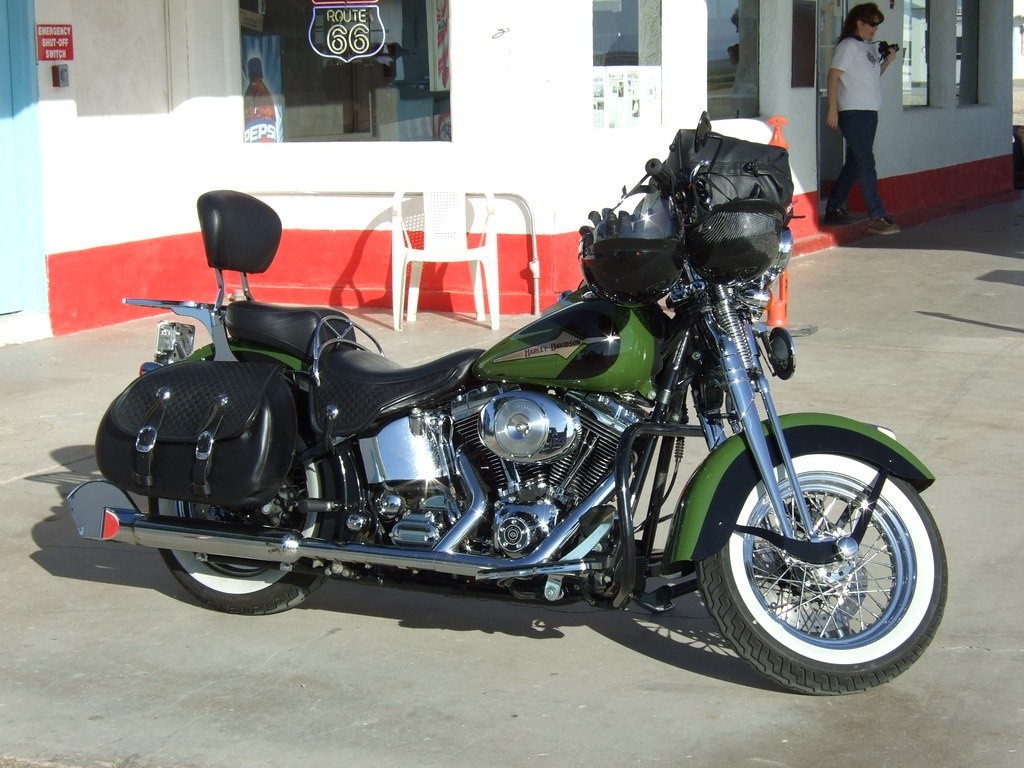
[878,41,899,59]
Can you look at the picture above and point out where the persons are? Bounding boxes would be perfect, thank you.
[824,3,901,235]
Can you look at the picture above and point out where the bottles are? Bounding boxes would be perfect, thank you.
[244,57,278,143]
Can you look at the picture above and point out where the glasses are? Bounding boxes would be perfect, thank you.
[865,20,880,27]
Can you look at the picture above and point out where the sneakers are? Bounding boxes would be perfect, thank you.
[824,208,855,224]
[867,217,902,235]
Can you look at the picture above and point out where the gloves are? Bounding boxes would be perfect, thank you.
[579,207,645,247]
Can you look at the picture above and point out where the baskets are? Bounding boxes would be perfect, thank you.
[681,198,787,286]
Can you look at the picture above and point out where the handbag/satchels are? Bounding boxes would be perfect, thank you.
[666,110,807,229]
[94,360,298,509]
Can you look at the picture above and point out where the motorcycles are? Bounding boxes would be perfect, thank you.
[63,110,950,698]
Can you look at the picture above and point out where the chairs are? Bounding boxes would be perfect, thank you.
[392,169,499,332]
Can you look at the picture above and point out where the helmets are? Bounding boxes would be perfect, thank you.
[577,233,685,309]
